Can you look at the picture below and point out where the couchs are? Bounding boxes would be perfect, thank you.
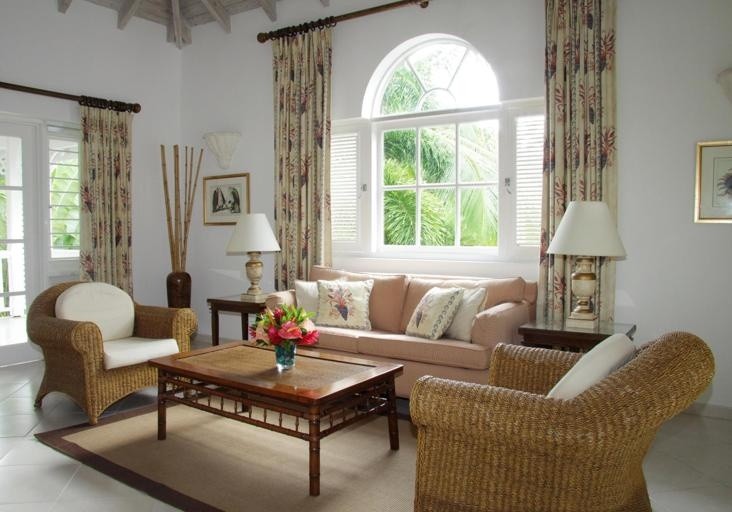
[22,277,201,432]
[264,261,540,408]
[408,329,716,510]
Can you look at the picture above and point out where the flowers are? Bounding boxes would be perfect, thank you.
[242,301,321,348]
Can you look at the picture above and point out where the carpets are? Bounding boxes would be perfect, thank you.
[31,382,416,512]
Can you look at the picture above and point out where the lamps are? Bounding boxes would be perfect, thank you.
[542,196,630,329]
[224,211,282,302]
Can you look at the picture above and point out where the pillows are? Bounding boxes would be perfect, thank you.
[316,275,376,334]
[404,286,467,342]
[309,264,411,334]
[445,284,491,343]
[398,275,527,336]
[291,274,353,327]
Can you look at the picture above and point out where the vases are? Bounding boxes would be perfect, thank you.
[165,270,192,310]
[268,341,300,370]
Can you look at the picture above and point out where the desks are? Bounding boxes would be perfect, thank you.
[204,290,275,346]
[519,316,638,356]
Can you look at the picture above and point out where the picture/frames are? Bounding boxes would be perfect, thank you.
[692,139,732,225]
[201,172,251,228]
